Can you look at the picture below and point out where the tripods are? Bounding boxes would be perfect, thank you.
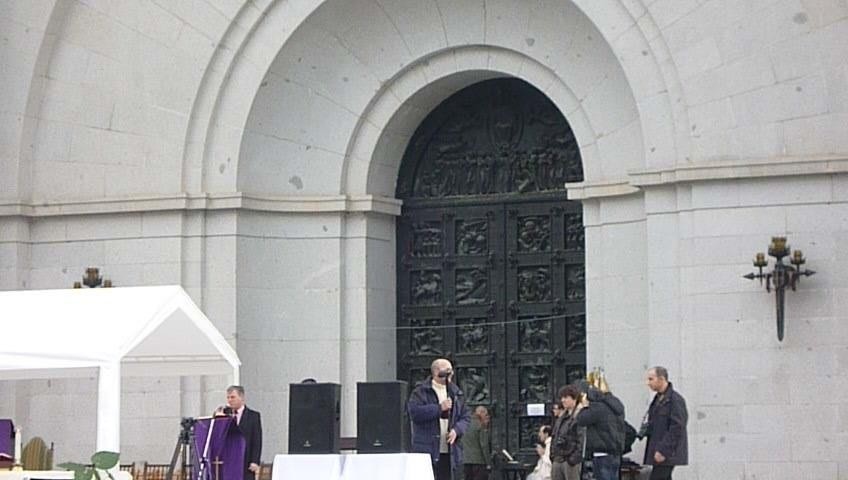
[166,431,204,480]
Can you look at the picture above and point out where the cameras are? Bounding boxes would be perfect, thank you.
[181,417,196,430]
[636,422,652,440]
[555,437,567,448]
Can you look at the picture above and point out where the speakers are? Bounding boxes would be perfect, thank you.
[355,381,412,453]
[288,383,341,453]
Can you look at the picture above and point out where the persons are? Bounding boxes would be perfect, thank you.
[223,386,262,480]
[457,405,493,480]
[642,366,688,480]
[406,359,471,480]
[526,378,625,480]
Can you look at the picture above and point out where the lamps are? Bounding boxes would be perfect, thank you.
[744,236,817,342]
